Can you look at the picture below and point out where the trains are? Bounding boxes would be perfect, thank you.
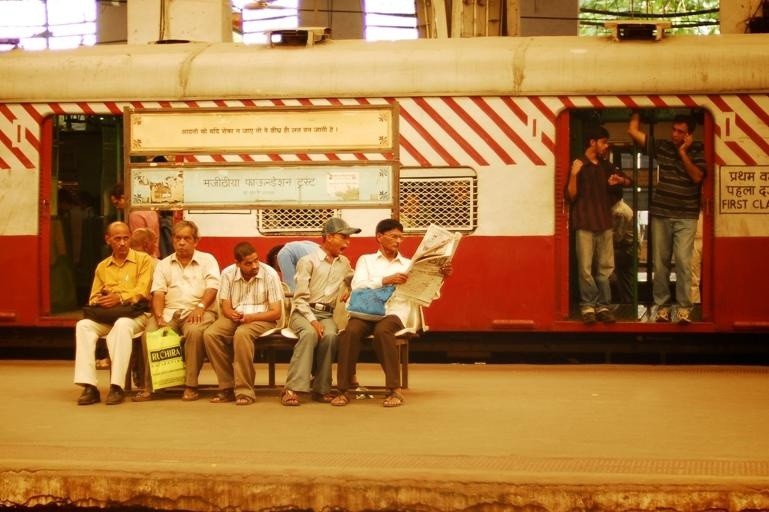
[1,18,769,366]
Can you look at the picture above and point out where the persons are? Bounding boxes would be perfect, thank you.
[330,218,454,408]
[202,240,285,405]
[564,107,709,325]
[266,240,321,288]
[130,220,221,402]
[156,210,175,260]
[72,221,156,406]
[281,216,363,406]
[94,227,160,371]
[108,181,160,259]
[399,182,477,230]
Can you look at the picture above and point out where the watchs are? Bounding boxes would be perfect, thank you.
[196,303,206,310]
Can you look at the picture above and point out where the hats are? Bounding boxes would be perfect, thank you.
[319,217,362,237]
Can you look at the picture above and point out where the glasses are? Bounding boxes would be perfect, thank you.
[381,233,404,242]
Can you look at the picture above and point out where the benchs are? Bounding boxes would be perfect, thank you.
[100,334,409,396]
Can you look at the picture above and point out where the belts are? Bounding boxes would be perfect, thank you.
[308,301,334,313]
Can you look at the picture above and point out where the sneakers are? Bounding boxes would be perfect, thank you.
[583,311,596,324]
[595,310,617,322]
[655,305,673,323]
[676,307,695,323]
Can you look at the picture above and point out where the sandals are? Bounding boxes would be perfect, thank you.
[280,390,299,407]
[183,387,200,401]
[210,389,236,403]
[236,393,255,405]
[132,390,162,402]
[383,391,406,407]
[312,389,358,409]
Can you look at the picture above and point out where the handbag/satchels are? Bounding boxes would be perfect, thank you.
[143,326,186,391]
[346,284,397,322]
[83,298,151,323]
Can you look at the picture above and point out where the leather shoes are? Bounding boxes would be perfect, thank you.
[105,384,125,405]
[76,386,101,404]
